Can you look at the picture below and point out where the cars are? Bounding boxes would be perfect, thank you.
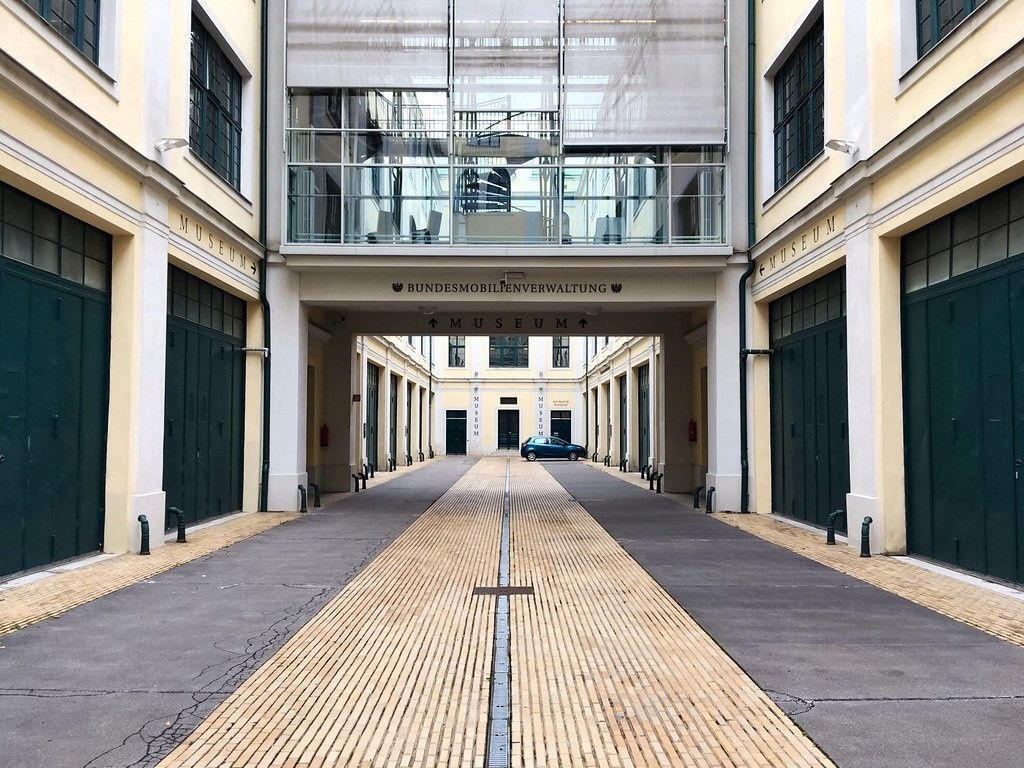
[520,435,587,462]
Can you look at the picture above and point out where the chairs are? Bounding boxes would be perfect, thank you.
[593,216,626,244]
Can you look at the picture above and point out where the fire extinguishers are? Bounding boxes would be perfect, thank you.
[689,416,697,440]
[320,422,329,446]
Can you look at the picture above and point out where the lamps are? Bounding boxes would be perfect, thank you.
[825,139,859,155]
[155,137,190,153]
